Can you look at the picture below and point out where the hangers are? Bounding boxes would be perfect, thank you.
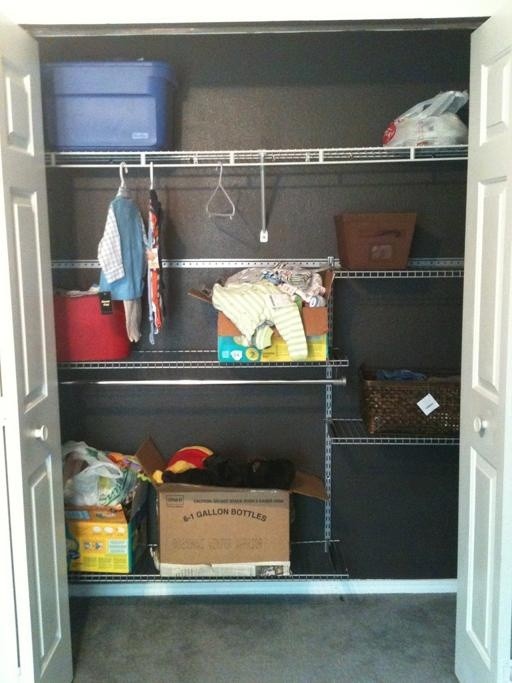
[205,161,235,221]
[118,161,129,188]
[149,162,154,190]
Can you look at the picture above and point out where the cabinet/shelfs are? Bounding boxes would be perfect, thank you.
[42,143,469,598]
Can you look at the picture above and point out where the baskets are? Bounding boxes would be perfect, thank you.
[357,365,459,438]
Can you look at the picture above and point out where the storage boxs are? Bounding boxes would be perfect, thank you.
[54,292,132,362]
[133,434,326,577]
[47,59,178,150]
[333,211,416,271]
[186,270,335,363]
[64,479,150,574]
